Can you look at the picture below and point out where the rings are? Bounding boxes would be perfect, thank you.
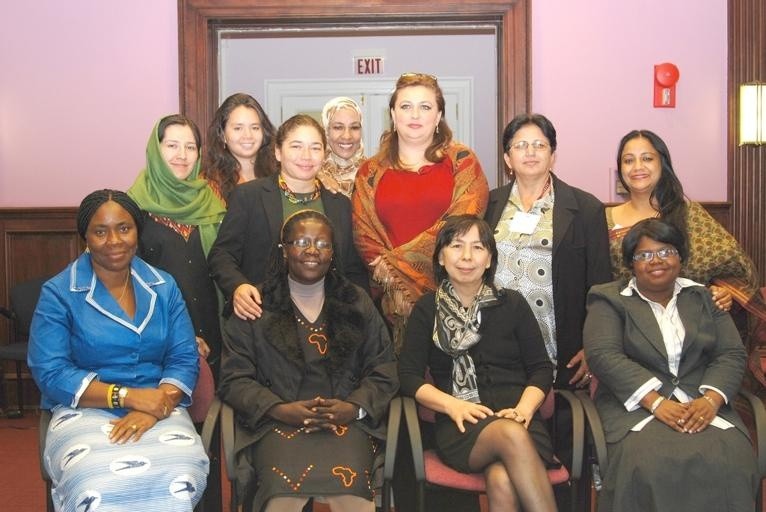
[676,419,682,425]
[699,416,705,421]
[585,372,593,379]
[130,424,137,431]
[513,411,518,416]
[163,407,169,418]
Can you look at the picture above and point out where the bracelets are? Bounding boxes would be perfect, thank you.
[649,395,665,414]
[702,394,719,412]
[107,383,121,409]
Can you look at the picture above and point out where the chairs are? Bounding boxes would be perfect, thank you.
[219,395,403,512]
[40,351,220,512]
[0,276,49,417]
[575,388,766,511]
[403,389,586,512]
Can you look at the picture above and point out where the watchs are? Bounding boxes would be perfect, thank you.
[356,407,367,421]
[119,386,128,409]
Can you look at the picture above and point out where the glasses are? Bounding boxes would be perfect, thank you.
[288,238,333,249]
[509,141,548,149]
[633,249,678,261]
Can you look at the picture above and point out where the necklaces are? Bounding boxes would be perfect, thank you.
[117,268,130,304]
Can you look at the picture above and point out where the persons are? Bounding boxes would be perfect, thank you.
[198,94,277,208]
[215,210,401,512]
[605,131,760,311]
[484,115,613,512]
[352,73,489,350]
[27,189,210,512]
[315,97,371,199]
[125,113,227,511]
[396,215,559,512]
[205,115,372,321]
[582,217,758,512]
